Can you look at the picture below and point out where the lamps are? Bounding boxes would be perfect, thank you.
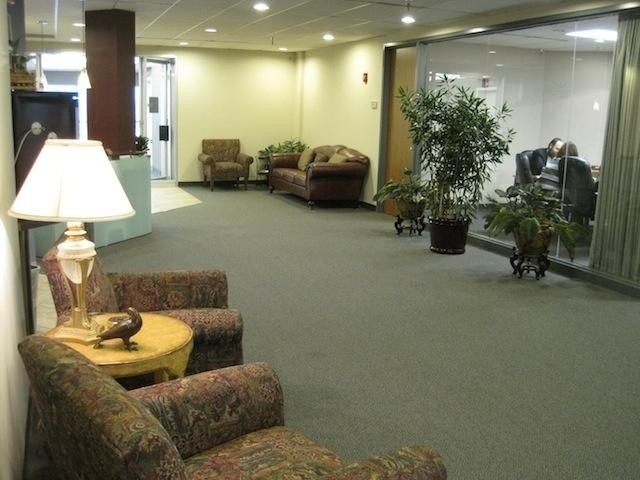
[8,136,135,344]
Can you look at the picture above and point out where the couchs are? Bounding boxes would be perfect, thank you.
[269,143,371,210]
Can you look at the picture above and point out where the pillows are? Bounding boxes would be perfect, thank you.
[298,148,347,170]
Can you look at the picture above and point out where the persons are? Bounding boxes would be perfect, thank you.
[531,138,598,222]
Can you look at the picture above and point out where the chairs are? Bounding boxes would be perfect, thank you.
[17,334,447,480]
[44,250,244,388]
[198,138,253,191]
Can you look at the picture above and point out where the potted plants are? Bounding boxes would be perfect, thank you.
[495,180,575,279]
[378,169,426,236]
[396,73,512,256]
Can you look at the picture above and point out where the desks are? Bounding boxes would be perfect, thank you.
[42,311,194,384]
